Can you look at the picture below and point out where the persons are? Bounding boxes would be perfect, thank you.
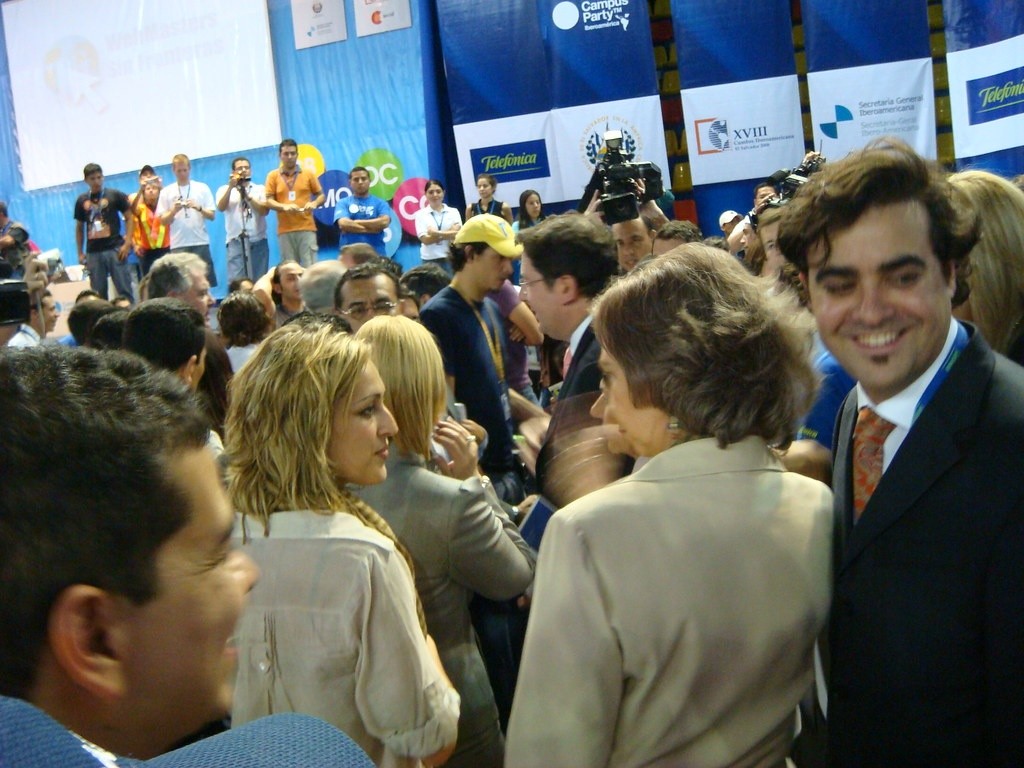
[333,166,391,257]
[264,140,326,267]
[0,152,1024,768]
[464,173,513,224]
[414,180,463,275]
[776,140,1024,768]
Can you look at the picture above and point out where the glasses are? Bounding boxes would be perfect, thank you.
[340,298,398,320]
[519,275,557,294]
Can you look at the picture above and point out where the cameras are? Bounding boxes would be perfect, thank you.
[236,177,251,185]
[298,208,305,214]
[144,179,155,184]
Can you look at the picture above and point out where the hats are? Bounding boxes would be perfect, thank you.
[719,210,743,227]
[139,164,155,176]
[453,213,524,257]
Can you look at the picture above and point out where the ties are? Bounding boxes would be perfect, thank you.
[852,406,897,524]
[563,348,573,380]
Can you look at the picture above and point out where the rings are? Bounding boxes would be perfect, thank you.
[468,435,476,442]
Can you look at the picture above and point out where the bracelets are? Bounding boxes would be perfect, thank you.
[481,475,490,487]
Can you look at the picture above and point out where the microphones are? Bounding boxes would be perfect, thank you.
[768,169,792,186]
